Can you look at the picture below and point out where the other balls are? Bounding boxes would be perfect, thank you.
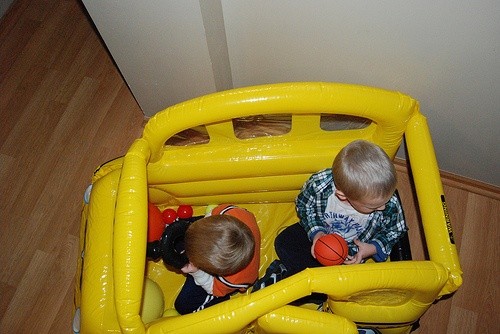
[314,233,349,266]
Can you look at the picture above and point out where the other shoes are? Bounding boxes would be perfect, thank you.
[146,241,162,261]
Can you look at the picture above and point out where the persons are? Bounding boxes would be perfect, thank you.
[174,203,260,317]
[251,138,405,311]
